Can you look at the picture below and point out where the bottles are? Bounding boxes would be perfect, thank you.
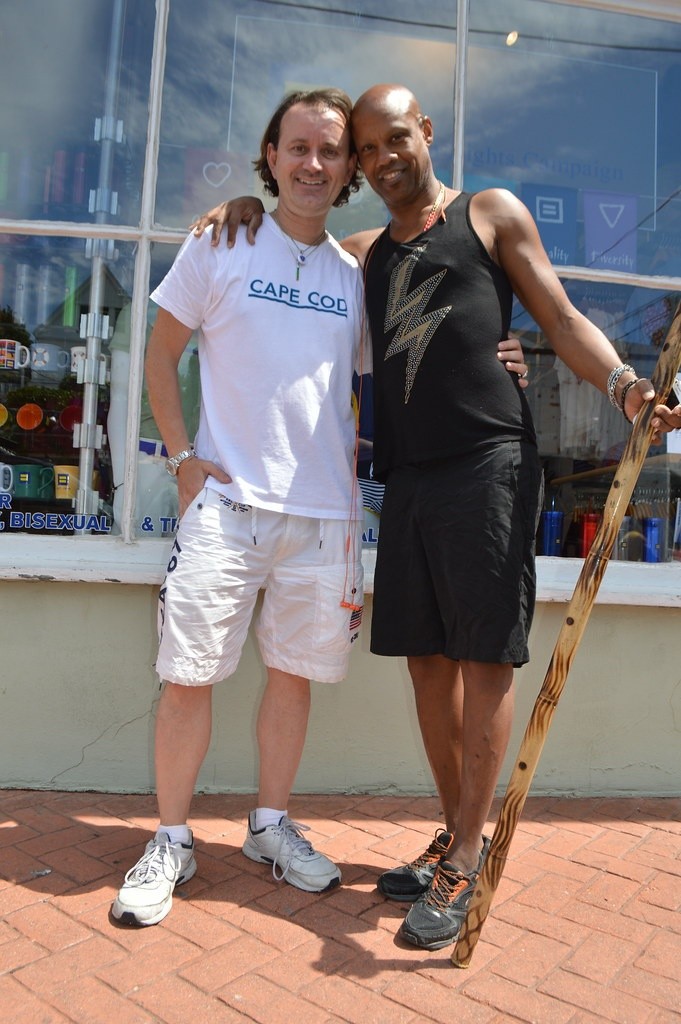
[12,263,81,329]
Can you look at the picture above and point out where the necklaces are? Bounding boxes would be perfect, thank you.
[274,209,325,282]
[424,180,445,231]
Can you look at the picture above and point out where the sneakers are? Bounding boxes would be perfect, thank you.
[401,833,491,951]
[111,829,197,927]
[241,809,342,891]
[376,828,457,900]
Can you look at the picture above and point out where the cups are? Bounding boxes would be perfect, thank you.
[0,461,101,501]
[0,401,83,434]
[0,338,86,378]
[539,510,563,557]
[642,517,664,563]
[580,513,602,557]
[611,516,631,561]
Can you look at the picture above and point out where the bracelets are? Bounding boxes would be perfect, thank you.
[607,364,639,424]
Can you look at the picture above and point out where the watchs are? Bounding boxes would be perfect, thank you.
[165,450,198,478]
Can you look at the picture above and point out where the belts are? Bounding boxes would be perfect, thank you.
[139,441,194,460]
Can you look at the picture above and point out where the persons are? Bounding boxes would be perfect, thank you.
[194,86,680,952]
[111,88,529,929]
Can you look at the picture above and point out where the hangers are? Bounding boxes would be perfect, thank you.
[576,284,630,316]
[570,485,677,527]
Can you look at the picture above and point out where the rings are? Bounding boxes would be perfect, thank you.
[518,370,528,378]
